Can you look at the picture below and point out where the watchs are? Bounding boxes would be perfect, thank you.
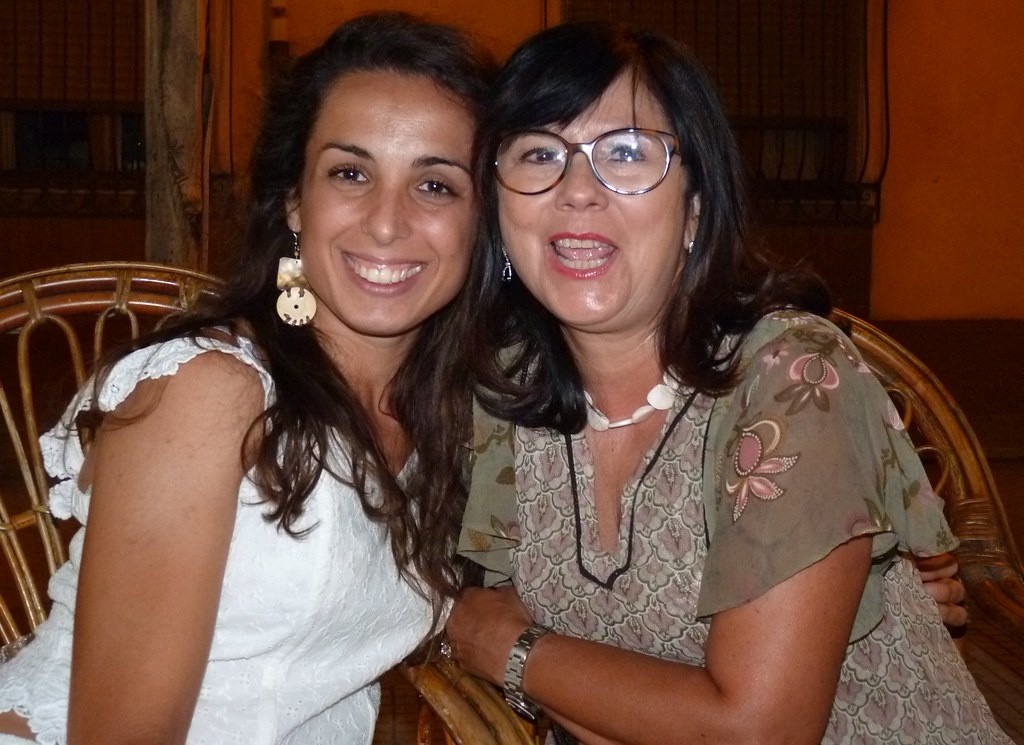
[504,623,558,722]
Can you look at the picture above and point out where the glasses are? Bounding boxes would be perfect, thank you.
[492,127,686,195]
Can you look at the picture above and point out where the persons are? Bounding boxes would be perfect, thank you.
[425,21,1021,745]
[0,12,971,745]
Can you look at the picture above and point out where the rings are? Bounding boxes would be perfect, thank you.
[439,641,452,656]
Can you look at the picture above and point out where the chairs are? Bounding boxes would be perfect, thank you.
[0,262,499,745]
[440,307,1024,745]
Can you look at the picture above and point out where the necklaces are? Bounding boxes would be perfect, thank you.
[584,364,688,430]
